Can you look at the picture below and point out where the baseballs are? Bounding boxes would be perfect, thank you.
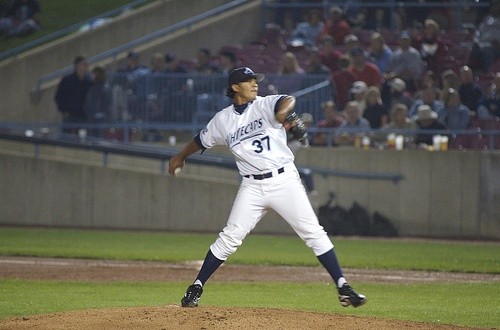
[173,168,183,175]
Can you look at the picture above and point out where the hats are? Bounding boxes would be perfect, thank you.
[350,80,368,93]
[228,66,265,86]
[389,78,407,90]
[412,105,437,121]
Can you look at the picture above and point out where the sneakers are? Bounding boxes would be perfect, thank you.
[181,284,203,307]
[338,290,367,307]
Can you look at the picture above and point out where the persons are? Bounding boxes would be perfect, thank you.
[168,66,366,307]
[0,0,42,38]
[56,0,500,149]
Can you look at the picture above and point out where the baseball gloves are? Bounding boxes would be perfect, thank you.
[287,113,309,145]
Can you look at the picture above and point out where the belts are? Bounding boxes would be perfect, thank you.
[245,167,284,180]
